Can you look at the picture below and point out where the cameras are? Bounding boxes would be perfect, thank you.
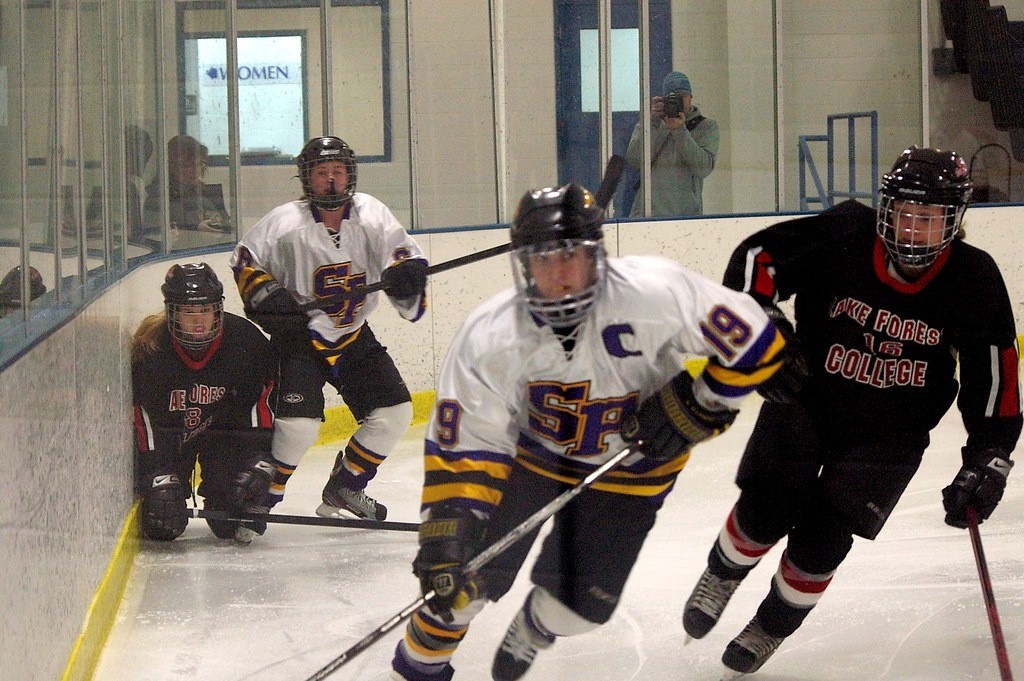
[661,93,684,118]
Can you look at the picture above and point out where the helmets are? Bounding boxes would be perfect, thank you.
[161,262,225,351]
[297,136,358,211]
[510,182,609,329]
[875,145,975,283]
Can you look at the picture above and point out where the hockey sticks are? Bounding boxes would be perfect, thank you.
[298,154,626,314]
[186,508,422,532]
[304,440,644,681]
[966,506,1014,680]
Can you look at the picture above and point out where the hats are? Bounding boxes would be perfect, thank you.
[662,71,693,99]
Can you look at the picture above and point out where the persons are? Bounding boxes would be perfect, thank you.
[683,146,1023,673]
[389,183,788,681]
[228,137,428,543]
[87,125,178,245]
[132,263,283,542]
[611,71,721,219]
[142,134,231,233]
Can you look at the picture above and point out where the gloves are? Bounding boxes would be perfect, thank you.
[146,473,192,541]
[411,511,481,624]
[619,370,739,461]
[231,459,277,506]
[756,346,822,408]
[244,279,310,337]
[942,446,1015,530]
[382,260,429,301]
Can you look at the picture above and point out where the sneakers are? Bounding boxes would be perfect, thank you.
[314,451,387,526]
[717,612,789,681]
[678,562,744,648]
[491,587,557,681]
[232,492,284,550]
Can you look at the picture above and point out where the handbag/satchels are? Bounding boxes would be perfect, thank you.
[611,161,643,220]
